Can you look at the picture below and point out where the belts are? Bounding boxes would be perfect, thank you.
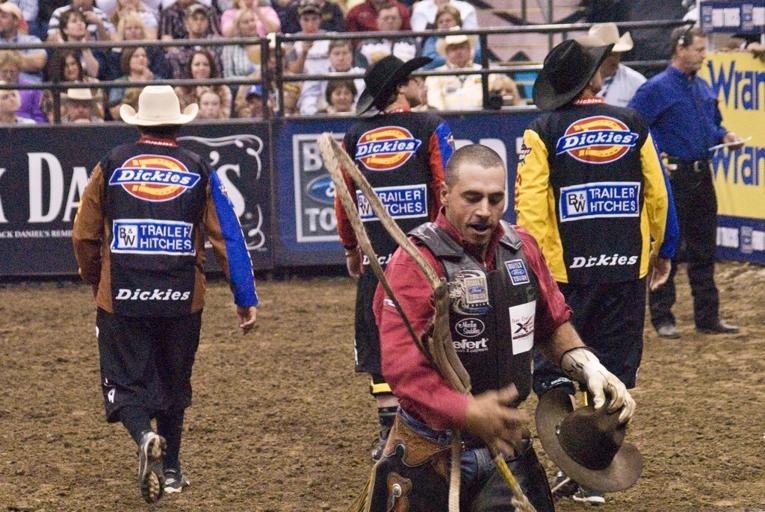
[670,158,707,175]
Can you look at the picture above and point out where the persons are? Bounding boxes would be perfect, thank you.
[577,27,648,104]
[334,54,466,462]
[71,85,259,506]
[192,89,231,119]
[345,0,410,46]
[164,0,220,40]
[285,5,338,74]
[320,81,358,117]
[173,53,232,117]
[358,8,419,62]
[220,1,280,36]
[47,0,114,44]
[299,39,368,117]
[174,7,230,78]
[237,54,301,117]
[426,30,496,109]
[1,50,47,123]
[1,82,37,124]
[421,5,482,67]
[485,75,521,109]
[54,86,103,125]
[515,40,678,504]
[48,8,108,81]
[110,0,158,26]
[220,12,265,79]
[241,85,283,116]
[39,52,105,125]
[113,23,168,75]
[2,2,46,73]
[108,48,167,119]
[282,0,348,35]
[368,145,635,512]
[628,26,743,338]
[413,1,480,36]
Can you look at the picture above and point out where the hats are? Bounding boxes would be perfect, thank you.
[535,381,643,493]
[186,3,207,15]
[443,34,467,49]
[297,0,320,15]
[118,85,199,127]
[530,38,615,111]
[573,21,633,53]
[355,54,433,117]
[0,2,23,22]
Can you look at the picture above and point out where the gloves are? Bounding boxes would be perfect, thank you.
[560,345,637,427]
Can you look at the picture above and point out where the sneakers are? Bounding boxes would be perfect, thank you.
[695,317,740,334]
[549,470,574,499]
[164,471,190,494]
[136,431,165,504]
[372,431,388,462]
[572,486,607,506]
[657,321,681,340]
